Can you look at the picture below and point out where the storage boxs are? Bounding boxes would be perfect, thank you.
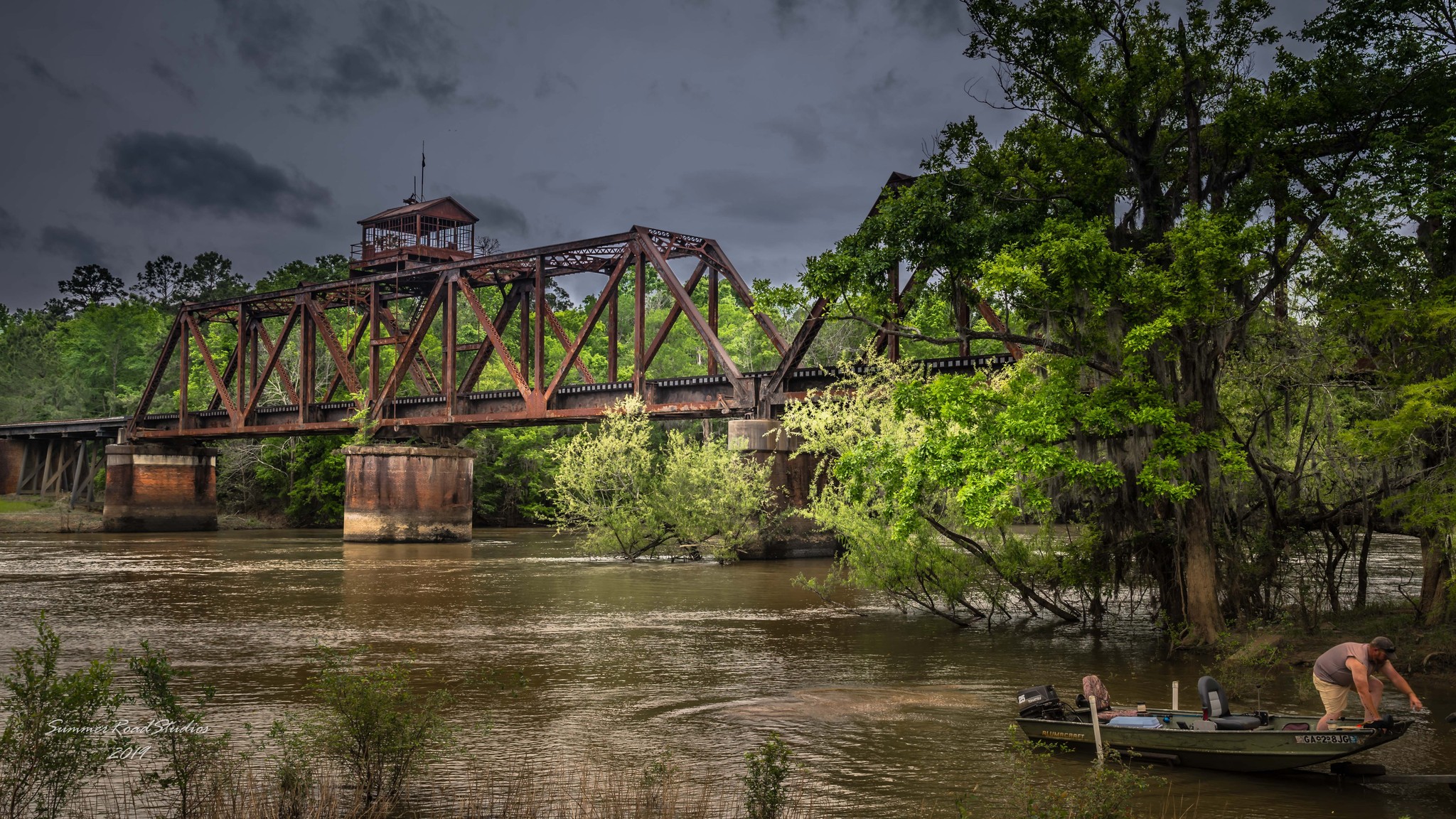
[1016,685,1059,715]
[1106,716,1163,728]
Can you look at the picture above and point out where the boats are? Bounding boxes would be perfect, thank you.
[1013,674,1415,776]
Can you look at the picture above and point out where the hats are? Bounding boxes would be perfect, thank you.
[1371,636,1398,660]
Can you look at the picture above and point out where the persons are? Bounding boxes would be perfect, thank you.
[1313,636,1422,731]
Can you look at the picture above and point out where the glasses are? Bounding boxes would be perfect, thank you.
[1375,645,1396,653]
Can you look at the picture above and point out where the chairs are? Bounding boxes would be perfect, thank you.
[1082,675,1138,722]
[1199,676,1261,730]
